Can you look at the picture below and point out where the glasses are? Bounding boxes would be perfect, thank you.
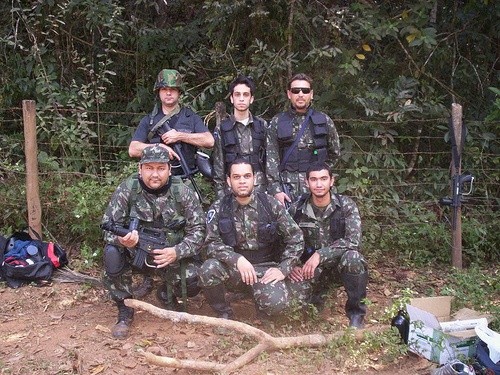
[291,88,311,94]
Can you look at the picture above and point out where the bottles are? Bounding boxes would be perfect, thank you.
[391,306,409,345]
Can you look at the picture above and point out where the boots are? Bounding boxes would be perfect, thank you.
[111,296,134,339]
[204,282,234,320]
[343,271,368,329]
[157,278,200,311]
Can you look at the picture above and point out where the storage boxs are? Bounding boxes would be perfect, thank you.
[407,296,494,363]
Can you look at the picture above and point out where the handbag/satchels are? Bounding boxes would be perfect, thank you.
[0,226,68,289]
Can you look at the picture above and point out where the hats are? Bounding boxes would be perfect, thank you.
[140,146,170,165]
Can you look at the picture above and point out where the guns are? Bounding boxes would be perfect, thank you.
[98,217,170,269]
[280,182,294,208]
[149,121,213,204]
[439,175,475,230]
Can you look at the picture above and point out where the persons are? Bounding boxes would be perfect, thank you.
[129,69,214,189]
[286,161,370,329]
[266,73,342,208]
[197,158,305,325]
[101,145,207,339]
[212,77,268,202]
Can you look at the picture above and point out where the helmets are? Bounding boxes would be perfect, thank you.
[154,69,185,92]
